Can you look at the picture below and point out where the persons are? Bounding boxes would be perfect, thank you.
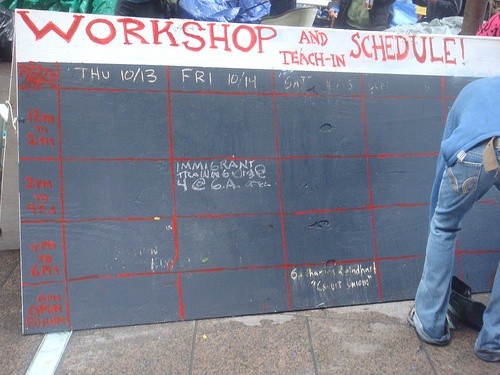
[408,75,500,363]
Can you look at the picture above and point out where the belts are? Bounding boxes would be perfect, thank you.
[480,137,500,171]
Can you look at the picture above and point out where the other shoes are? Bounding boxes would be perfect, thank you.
[407,304,451,344]
[473,337,500,361]
